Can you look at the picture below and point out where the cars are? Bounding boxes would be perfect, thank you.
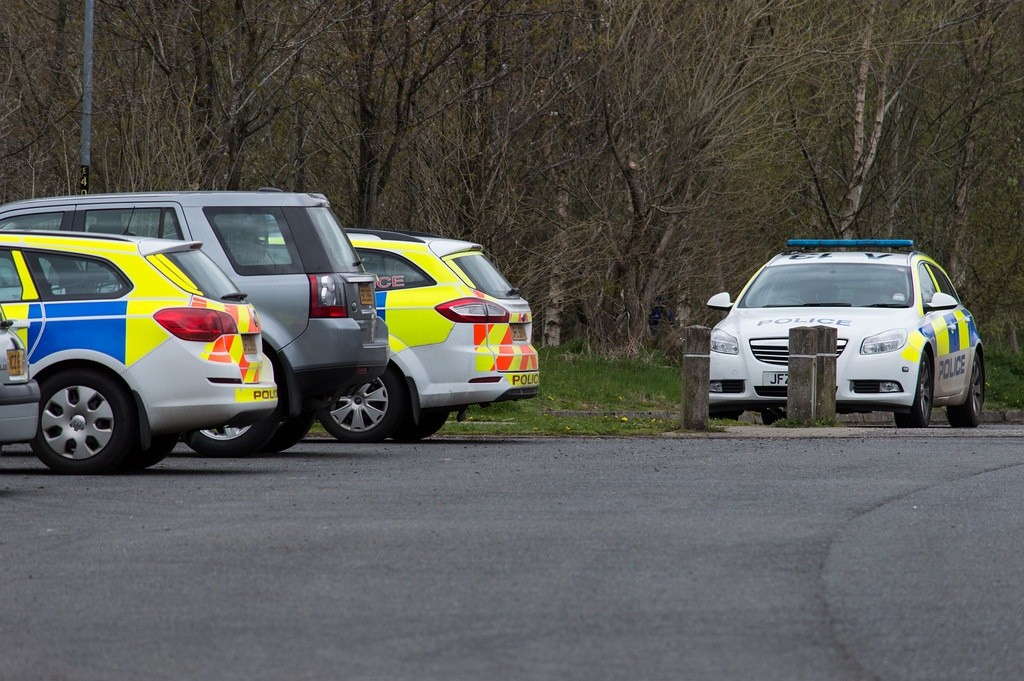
[0,223,281,475]
[262,225,539,443]
[701,238,986,427]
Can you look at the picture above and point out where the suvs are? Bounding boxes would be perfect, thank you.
[0,187,392,457]
